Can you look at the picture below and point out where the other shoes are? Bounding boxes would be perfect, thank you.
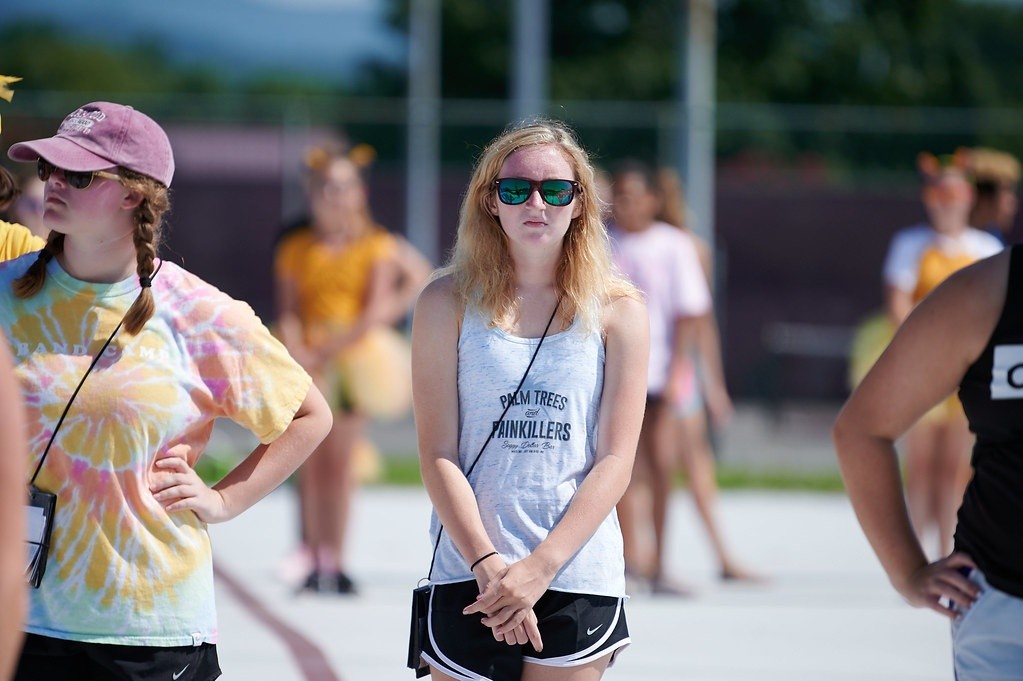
[334,571,354,595]
[304,569,320,592]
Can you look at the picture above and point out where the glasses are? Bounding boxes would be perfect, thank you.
[37,155,123,190]
[491,177,581,207]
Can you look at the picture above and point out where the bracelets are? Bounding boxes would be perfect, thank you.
[471,551,497,570]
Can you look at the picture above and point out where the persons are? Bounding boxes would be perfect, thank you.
[405,125,652,681]
[831,246,1023,681]
[882,149,1005,560]
[272,156,434,596]
[0,100,333,680]
[607,158,711,596]
[0,167,44,263]
[647,162,748,596]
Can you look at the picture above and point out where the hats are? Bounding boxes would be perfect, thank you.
[7,102,175,189]
